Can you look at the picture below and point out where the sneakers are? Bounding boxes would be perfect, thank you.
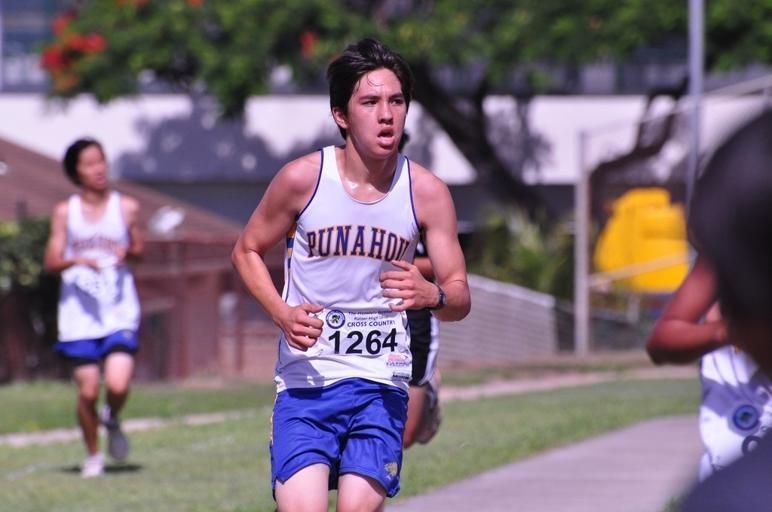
[415,368,441,444]
[80,452,107,479]
[99,405,130,460]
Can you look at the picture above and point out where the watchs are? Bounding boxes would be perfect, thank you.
[426,284,446,310]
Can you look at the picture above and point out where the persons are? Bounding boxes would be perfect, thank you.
[44,141,144,477]
[404,238,440,448]
[231,41,471,512]
[646,254,772,481]
[661,107,772,512]
